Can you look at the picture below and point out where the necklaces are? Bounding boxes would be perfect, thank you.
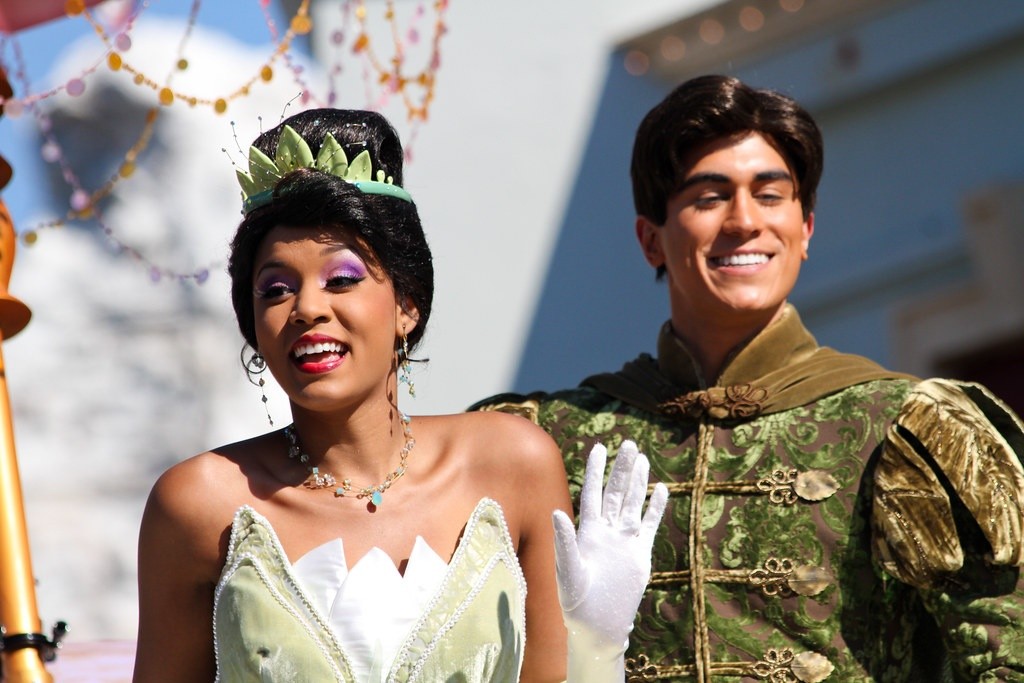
[284,407,416,506]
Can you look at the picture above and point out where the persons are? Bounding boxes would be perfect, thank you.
[130,108,669,683]
[468,74,1024,683]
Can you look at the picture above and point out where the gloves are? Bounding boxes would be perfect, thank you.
[552,439,669,683]
